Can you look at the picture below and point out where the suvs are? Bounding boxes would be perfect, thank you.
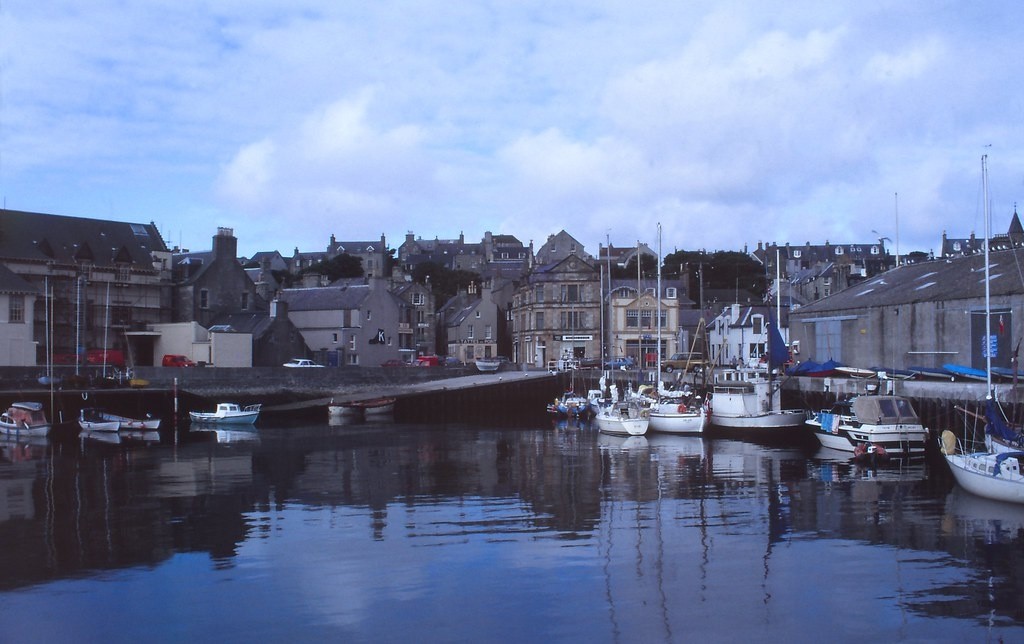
[656,350,710,373]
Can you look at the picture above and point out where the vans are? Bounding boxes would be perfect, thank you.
[162,355,195,366]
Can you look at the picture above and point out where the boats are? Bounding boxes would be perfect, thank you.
[189,402,263,425]
[92,410,162,429]
[0,400,53,437]
[327,395,363,417]
[361,397,397,415]
[544,220,1024,454]
[804,342,929,461]
[77,406,120,432]
[475,356,502,373]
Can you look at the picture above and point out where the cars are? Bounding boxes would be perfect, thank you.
[601,357,634,370]
[379,354,463,367]
[282,358,326,368]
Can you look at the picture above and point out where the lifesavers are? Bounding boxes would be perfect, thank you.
[677,404,687,413]
[639,410,650,419]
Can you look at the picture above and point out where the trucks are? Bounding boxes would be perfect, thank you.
[53,344,127,366]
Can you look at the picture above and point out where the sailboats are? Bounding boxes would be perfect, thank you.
[35,275,152,390]
[934,155,1023,507]
